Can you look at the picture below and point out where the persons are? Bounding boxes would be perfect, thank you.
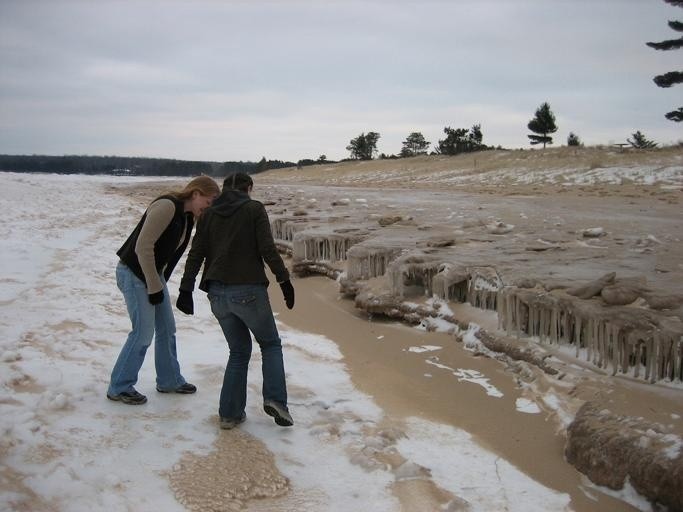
[105,176,221,404]
[174,172,297,431]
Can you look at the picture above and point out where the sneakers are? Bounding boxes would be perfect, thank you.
[263,399,294,426]
[106,390,147,405]
[156,381,196,394]
[219,411,246,430]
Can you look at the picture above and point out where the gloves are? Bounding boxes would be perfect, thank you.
[176,288,194,315]
[280,279,295,310]
[148,289,165,306]
